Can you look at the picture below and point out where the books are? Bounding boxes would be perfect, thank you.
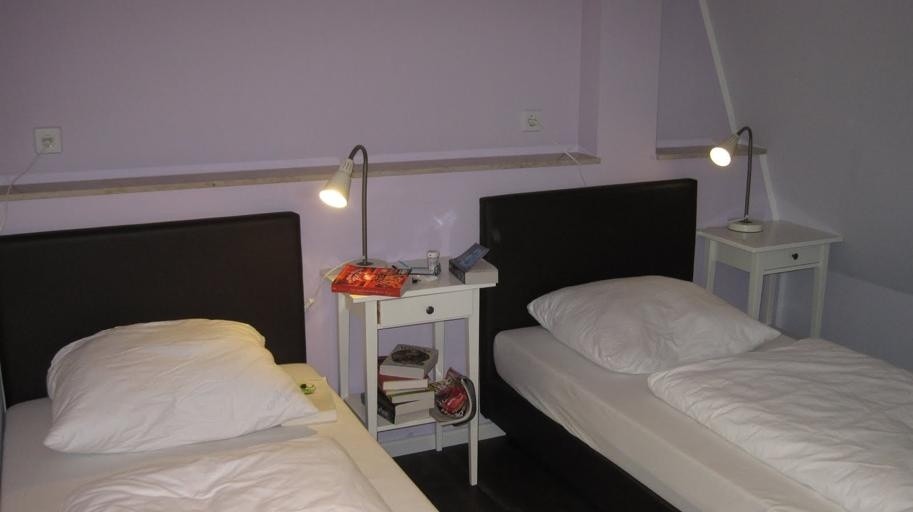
[449,243,499,285]
[361,343,439,424]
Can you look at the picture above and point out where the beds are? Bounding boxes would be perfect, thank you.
[479,176,913,511]
[1,212,445,512]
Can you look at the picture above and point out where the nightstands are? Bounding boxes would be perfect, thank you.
[697,218,844,342]
[318,258,496,489]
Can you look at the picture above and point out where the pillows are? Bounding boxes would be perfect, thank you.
[38,316,319,454]
[527,273,782,377]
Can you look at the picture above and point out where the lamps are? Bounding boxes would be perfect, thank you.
[320,146,390,272]
[708,126,766,235]
